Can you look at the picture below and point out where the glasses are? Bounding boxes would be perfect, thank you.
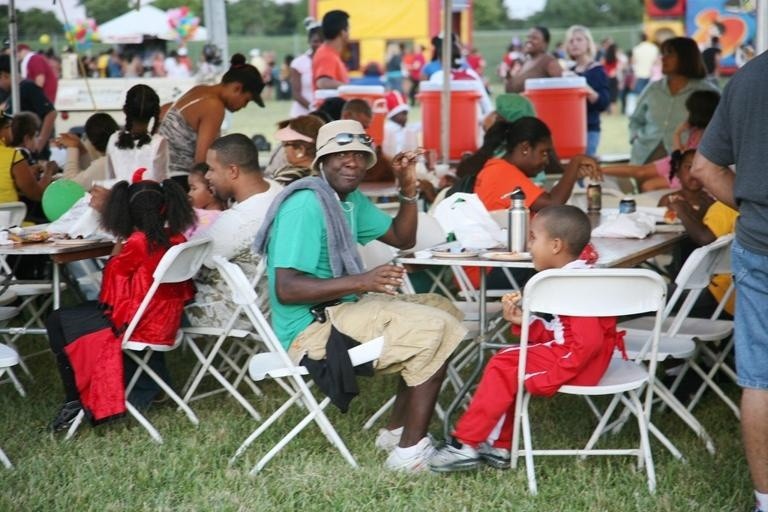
[318,133,374,146]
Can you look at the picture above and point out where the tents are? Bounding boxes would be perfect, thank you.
[96,4,208,44]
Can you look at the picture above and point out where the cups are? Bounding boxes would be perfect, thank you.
[433,160,450,177]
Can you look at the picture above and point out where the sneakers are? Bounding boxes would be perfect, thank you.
[385,437,437,470]
[428,442,480,471]
[154,386,171,407]
[479,440,510,469]
[375,424,436,448]
[48,398,87,431]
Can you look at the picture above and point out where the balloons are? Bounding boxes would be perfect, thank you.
[41,178,84,221]
[63,18,103,53]
[39,33,51,45]
[167,5,200,47]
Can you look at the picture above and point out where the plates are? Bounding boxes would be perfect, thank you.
[48,235,106,245]
[479,252,532,261]
[431,248,488,257]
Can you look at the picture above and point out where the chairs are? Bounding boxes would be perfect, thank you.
[63,238,212,444]
[177,259,266,423]
[510,269,669,497]
[614,248,740,423]
[212,256,387,475]
[0,343,25,399]
[397,212,497,392]
[580,234,734,466]
[364,236,470,437]
[0,307,31,386]
[0,259,67,382]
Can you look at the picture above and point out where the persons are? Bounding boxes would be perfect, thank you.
[87,132,285,404]
[417,174,456,204]
[53,111,121,192]
[268,114,325,189]
[658,148,716,210]
[97,52,109,78]
[177,46,193,70]
[665,194,741,322]
[382,89,411,161]
[447,120,511,198]
[0,54,57,160]
[505,26,562,94]
[106,49,121,78]
[406,44,426,107]
[429,43,492,148]
[311,9,349,108]
[163,49,178,77]
[46,178,196,436]
[688,49,768,512]
[9,112,40,166]
[105,84,171,185]
[563,25,613,188]
[384,42,408,103]
[500,37,523,76]
[266,119,469,474]
[250,49,268,77]
[279,55,293,81]
[17,45,58,104]
[523,42,532,61]
[0,141,58,227]
[602,44,619,113]
[597,38,612,59]
[632,34,659,92]
[428,203,629,473]
[288,16,322,117]
[158,53,266,174]
[628,37,722,165]
[164,162,229,242]
[340,99,384,181]
[349,63,383,85]
[452,115,601,291]
[599,90,721,193]
[121,45,143,79]
[466,48,492,94]
[82,56,93,78]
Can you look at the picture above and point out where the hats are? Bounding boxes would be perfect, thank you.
[275,123,313,143]
[232,68,265,109]
[310,119,377,175]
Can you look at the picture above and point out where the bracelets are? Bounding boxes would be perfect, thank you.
[398,190,420,203]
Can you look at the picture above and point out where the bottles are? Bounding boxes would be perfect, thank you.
[587,170,601,211]
[620,197,636,214]
[508,187,528,253]
